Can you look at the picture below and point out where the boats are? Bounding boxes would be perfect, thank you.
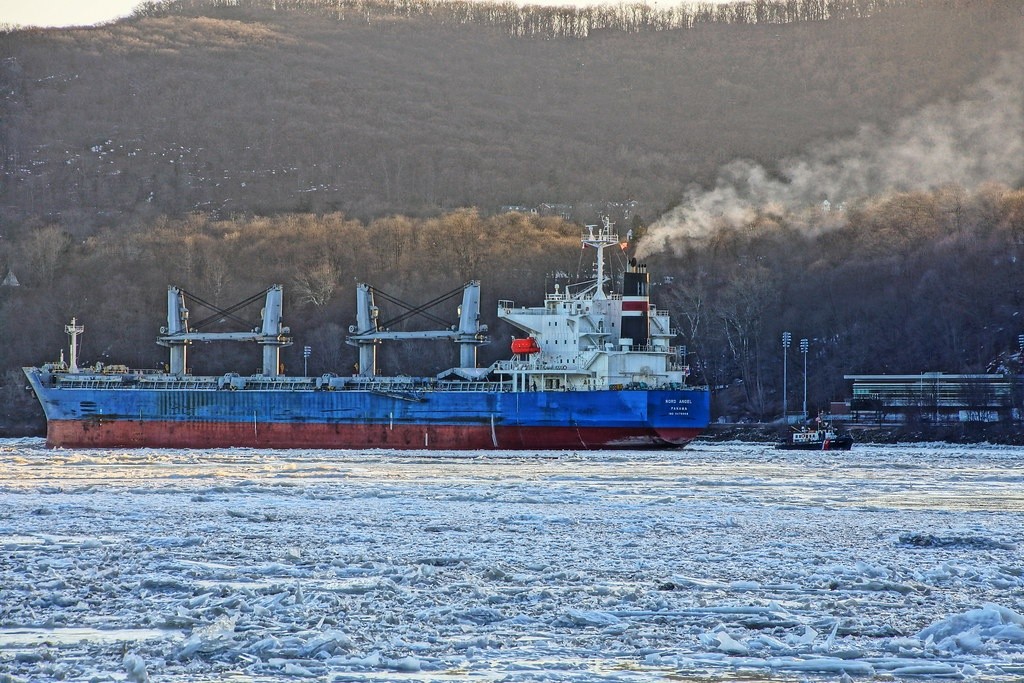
[509,336,541,354]
[20,217,714,452]
[775,408,868,454]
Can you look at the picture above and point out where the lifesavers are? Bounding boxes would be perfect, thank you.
[506,309,511,314]
[563,365,567,370]
[585,306,589,311]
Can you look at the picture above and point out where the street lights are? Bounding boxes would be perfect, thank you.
[782,331,792,415]
[799,338,808,422]
[304,345,311,377]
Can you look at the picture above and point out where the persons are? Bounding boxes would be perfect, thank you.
[633,385,637,390]
[532,381,537,392]
[662,383,665,390]
[103,353,111,366]
[622,384,629,391]
[96,354,102,372]
[566,388,570,392]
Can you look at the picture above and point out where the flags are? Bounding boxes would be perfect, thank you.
[686,366,690,377]
[584,243,589,248]
